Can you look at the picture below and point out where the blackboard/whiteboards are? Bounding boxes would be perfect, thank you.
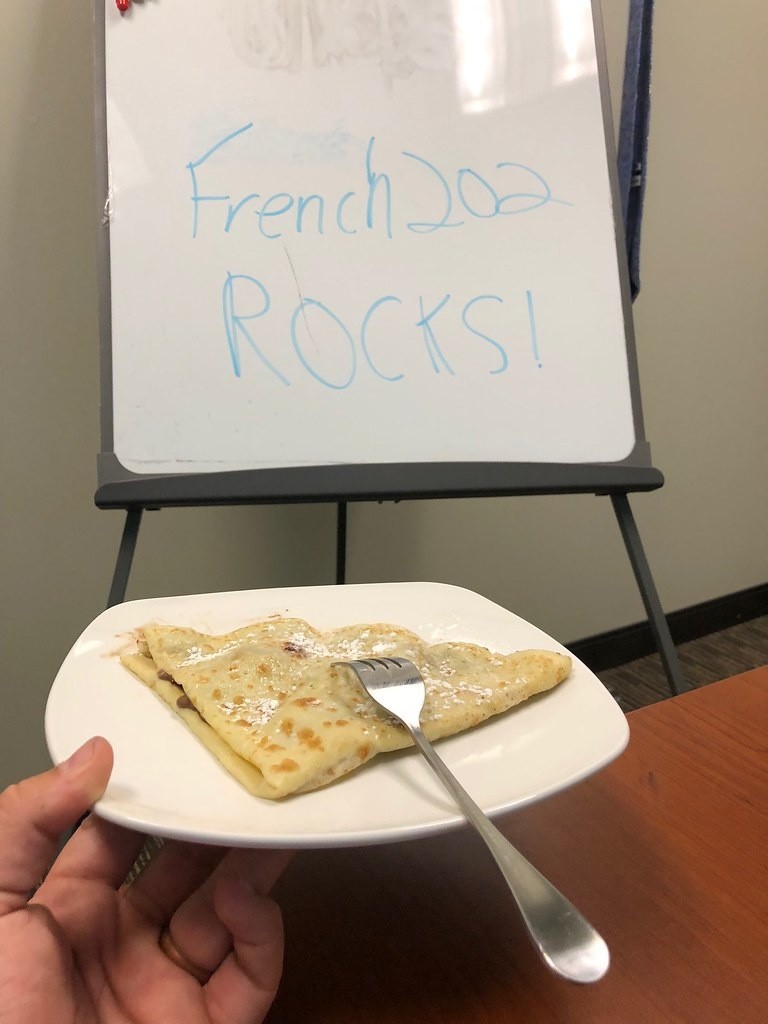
[90,2,667,510]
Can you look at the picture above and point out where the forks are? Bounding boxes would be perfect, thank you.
[330,657,611,985]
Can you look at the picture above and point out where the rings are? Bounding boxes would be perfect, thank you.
[160,927,212,983]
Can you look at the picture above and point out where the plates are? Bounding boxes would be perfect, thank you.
[45,581,630,848]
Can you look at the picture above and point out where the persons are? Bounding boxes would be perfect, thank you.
[0,736,298,1024]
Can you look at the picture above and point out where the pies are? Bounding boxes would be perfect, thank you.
[120,617,573,800]
